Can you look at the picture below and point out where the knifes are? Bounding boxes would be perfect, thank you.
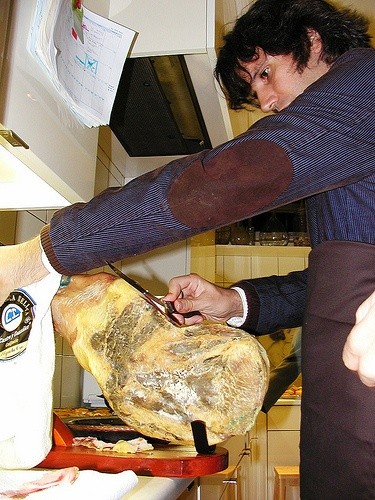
[109,261,204,318]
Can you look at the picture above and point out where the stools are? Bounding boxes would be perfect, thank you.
[273,465,300,500]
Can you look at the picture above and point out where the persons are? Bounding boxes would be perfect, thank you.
[342,293,375,387]
[0,0,375,500]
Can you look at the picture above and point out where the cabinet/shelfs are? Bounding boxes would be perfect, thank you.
[178,406,301,500]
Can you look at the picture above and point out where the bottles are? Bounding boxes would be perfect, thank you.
[260,210,290,246]
[230,220,251,245]
[293,206,311,247]
